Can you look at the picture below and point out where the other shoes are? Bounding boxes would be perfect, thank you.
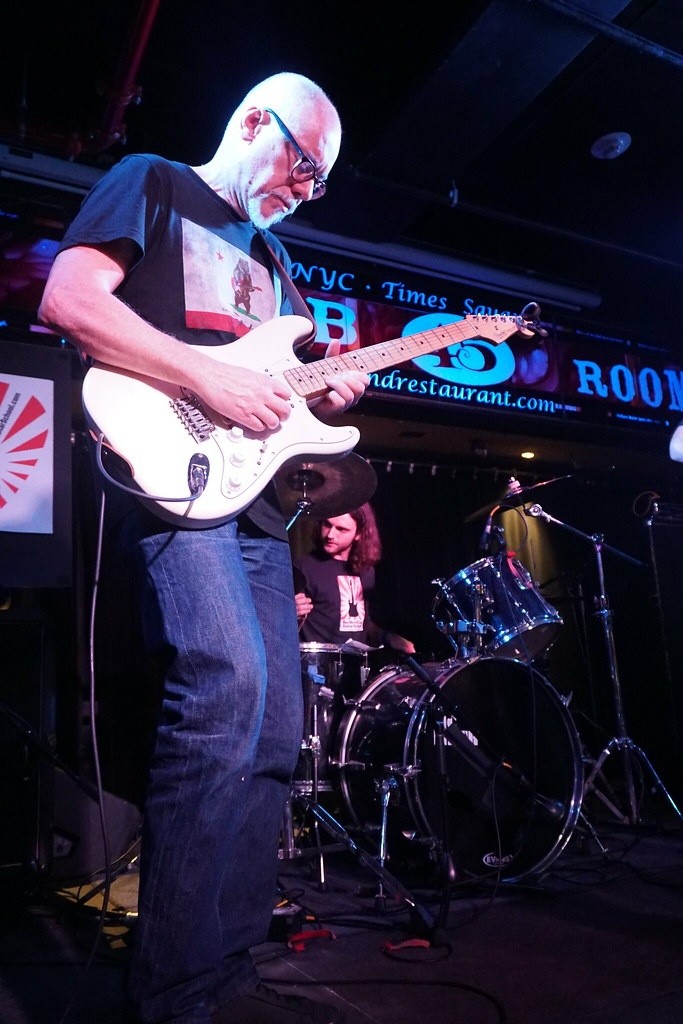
[211,983,345,1024]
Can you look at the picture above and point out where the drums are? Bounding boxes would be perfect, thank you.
[339,658,591,881]
[293,639,344,746]
[291,750,332,798]
[427,550,563,664]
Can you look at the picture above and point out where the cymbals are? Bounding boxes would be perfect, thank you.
[452,475,584,519]
[265,454,378,521]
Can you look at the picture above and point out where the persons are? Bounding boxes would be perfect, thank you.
[294,502,416,688]
[38,72,370,1024]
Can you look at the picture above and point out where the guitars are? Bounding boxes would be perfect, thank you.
[78,310,553,533]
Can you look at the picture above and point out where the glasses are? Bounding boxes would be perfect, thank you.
[262,107,326,200]
